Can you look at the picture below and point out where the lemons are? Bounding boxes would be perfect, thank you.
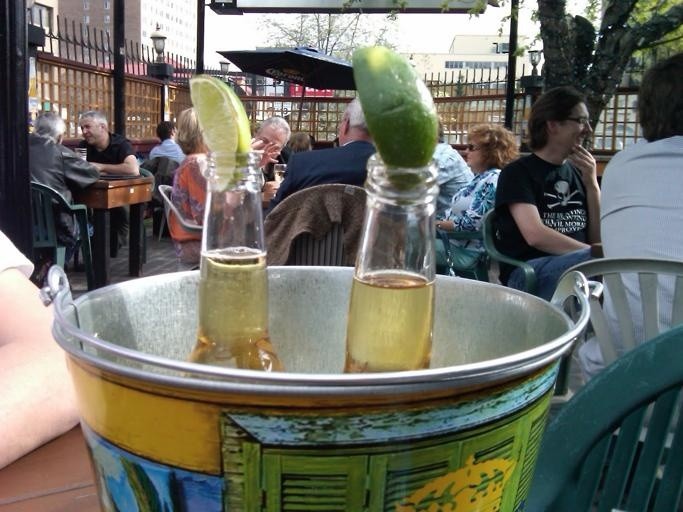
[353,45,440,206]
[188,74,252,195]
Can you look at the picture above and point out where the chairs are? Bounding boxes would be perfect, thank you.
[263,183,368,267]
[158,185,202,270]
[433,231,488,282]
[140,157,180,241]
[139,168,155,193]
[523,323,683,512]
[29,182,95,291]
[555,259,683,395]
[482,208,536,297]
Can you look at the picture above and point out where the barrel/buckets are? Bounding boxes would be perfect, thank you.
[37,264,604,512]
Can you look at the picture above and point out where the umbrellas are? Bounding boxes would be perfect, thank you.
[216,45,356,133]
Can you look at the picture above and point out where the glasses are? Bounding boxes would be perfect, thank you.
[468,144,479,151]
[567,116,587,124]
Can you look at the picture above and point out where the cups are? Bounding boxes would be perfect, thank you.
[273,163,286,184]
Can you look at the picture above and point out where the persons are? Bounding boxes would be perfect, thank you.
[492,83,601,310]
[169,107,378,272]
[28,110,139,269]
[578,53,682,432]
[431,118,520,271]
[1,228,84,472]
[149,122,185,166]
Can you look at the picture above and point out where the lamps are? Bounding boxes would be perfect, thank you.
[527,40,544,76]
[147,22,173,78]
[219,62,231,83]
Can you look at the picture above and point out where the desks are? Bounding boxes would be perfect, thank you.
[262,181,282,210]
[66,176,154,289]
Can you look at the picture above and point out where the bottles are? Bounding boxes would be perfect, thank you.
[341,152,440,374]
[189,150,280,373]
